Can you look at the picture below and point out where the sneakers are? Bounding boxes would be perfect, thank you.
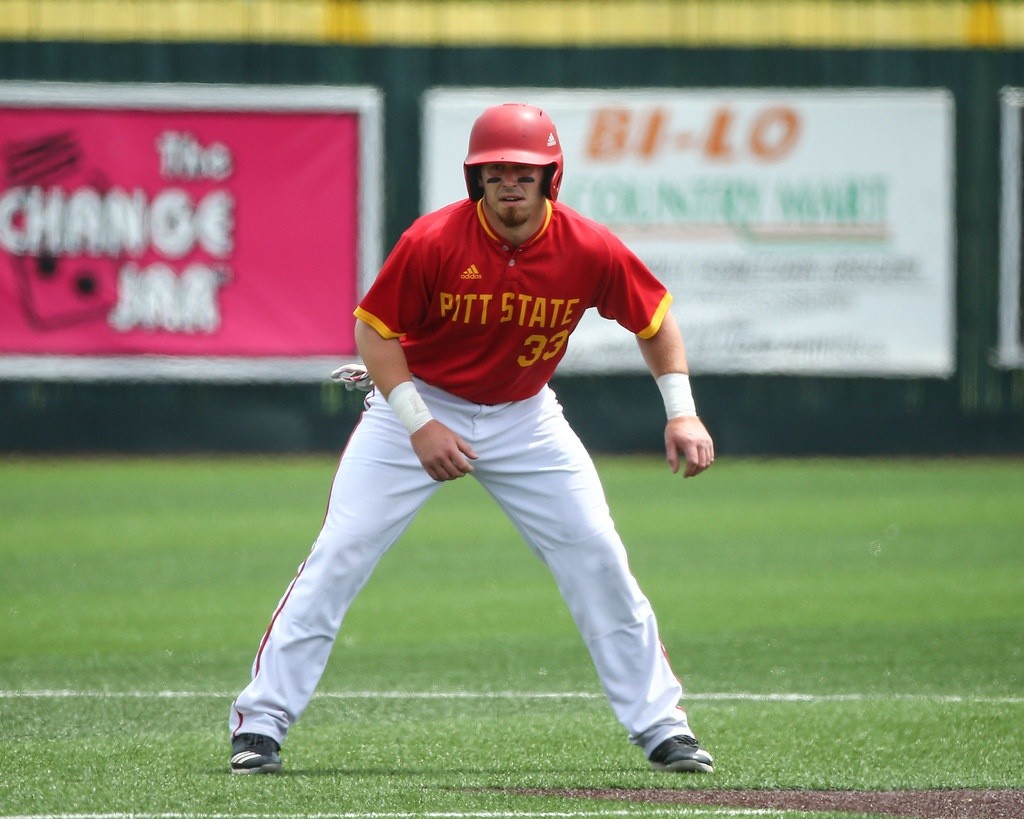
[230,732,282,774]
[647,734,713,773]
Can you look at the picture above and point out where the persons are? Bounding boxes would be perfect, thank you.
[230,104,716,774]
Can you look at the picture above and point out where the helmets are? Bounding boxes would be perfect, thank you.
[464,104,564,204]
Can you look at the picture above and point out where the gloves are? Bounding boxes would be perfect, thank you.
[331,364,375,392]
